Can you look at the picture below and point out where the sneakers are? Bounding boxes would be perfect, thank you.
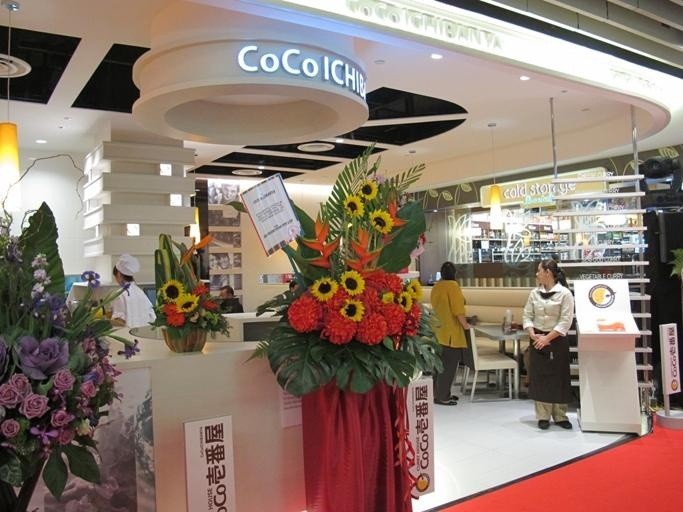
[554,421,572,428]
[538,420,549,428]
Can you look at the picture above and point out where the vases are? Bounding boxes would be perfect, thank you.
[162,329,207,353]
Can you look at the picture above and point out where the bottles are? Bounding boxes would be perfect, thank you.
[501,308,514,336]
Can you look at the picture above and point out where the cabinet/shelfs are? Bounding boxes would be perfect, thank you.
[472,238,569,263]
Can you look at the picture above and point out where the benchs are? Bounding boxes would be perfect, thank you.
[422,285,536,375]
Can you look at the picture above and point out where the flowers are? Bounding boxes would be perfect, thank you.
[242,142,445,397]
[149,234,233,340]
[0,152,140,512]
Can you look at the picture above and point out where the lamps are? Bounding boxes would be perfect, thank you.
[487,122,503,230]
[1,0,20,181]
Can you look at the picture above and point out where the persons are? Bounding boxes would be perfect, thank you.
[109,253,157,329]
[430,262,471,405]
[218,285,244,314]
[289,281,298,293]
[208,182,241,289]
[522,259,575,430]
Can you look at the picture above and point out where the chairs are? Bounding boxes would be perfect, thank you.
[461,327,517,402]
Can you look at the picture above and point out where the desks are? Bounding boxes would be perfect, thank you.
[473,326,530,400]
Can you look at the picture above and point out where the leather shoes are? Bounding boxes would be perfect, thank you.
[434,395,458,405]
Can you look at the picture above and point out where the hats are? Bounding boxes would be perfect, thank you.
[115,253,140,276]
